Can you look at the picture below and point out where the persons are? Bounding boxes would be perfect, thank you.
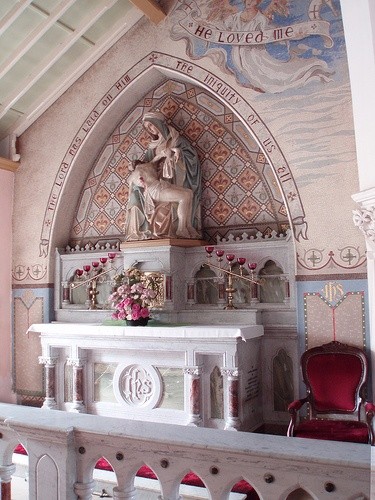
[124,111,202,239]
[129,158,200,237]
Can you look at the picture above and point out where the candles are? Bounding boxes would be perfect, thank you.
[229,275,232,286]
[91,280,96,288]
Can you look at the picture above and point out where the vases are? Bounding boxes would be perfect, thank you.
[126,318,149,326]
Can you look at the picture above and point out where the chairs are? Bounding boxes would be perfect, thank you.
[288,339,375,445]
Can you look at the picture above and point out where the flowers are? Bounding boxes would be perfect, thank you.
[108,282,158,320]
